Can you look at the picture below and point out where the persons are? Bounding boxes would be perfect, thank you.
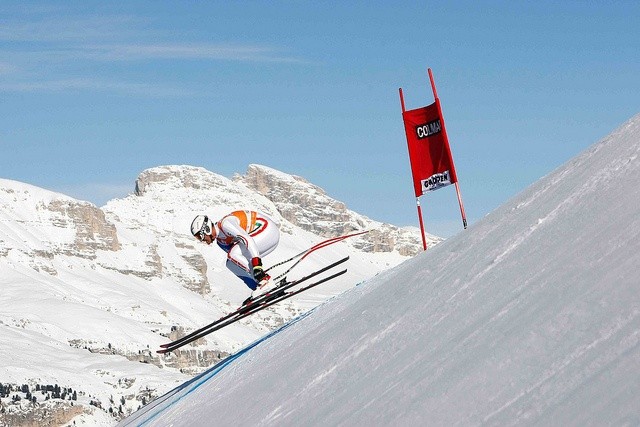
[191,210,280,300]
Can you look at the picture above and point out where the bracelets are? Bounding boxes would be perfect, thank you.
[252,257,262,267]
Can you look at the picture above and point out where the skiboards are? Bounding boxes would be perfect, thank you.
[156,251,349,355]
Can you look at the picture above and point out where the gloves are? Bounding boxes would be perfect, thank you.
[253,268,265,278]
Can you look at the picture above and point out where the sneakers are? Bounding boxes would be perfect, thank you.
[251,276,276,298]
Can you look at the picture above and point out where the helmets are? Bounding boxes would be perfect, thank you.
[190,215,212,240]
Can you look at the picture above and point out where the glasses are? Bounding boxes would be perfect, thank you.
[194,215,208,240]
